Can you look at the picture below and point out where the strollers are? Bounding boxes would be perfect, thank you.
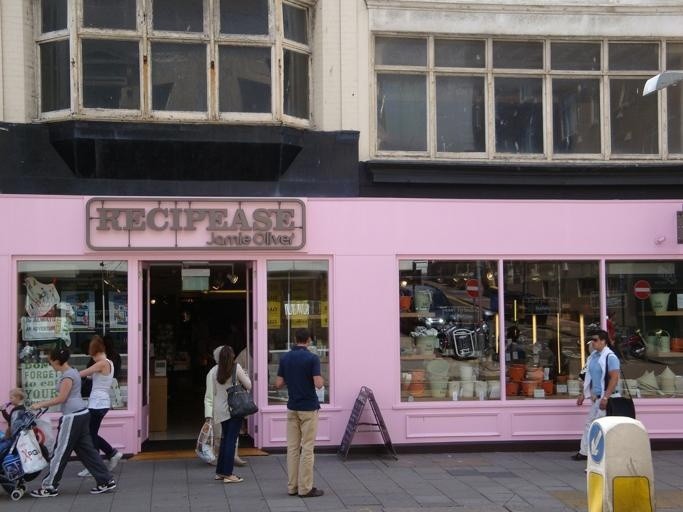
[0,405,50,502]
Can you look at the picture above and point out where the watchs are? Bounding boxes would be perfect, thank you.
[602,396,609,402]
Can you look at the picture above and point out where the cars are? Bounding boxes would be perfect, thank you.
[490,290,549,326]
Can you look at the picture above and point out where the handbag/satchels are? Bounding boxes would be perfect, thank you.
[194,417,217,465]
[605,393,636,419]
[81,376,93,397]
[16,426,49,474]
[226,362,259,422]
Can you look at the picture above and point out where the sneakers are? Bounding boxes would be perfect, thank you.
[234,455,247,465]
[88,479,117,494]
[29,487,59,498]
[107,450,124,472]
[77,467,95,477]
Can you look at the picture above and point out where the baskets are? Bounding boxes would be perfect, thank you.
[2,454,25,482]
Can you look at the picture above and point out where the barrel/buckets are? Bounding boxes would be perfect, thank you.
[649,290,670,312]
[673,337,682,352]
[505,363,543,396]
[657,329,671,353]
[428,359,451,398]
[542,380,553,395]
[568,380,580,396]
[414,289,432,311]
[399,295,412,310]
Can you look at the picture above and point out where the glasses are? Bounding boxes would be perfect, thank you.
[590,337,598,342]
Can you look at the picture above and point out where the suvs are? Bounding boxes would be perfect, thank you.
[399,283,463,340]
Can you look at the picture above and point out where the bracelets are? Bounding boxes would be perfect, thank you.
[38,402,41,408]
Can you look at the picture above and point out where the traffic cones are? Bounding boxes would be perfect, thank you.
[465,279,483,297]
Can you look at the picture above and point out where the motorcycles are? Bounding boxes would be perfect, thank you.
[579,316,646,361]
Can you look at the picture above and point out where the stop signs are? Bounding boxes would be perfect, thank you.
[633,279,650,299]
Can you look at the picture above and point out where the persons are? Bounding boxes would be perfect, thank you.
[570,329,622,461]
[213,346,252,484]
[275,327,324,498]
[30,348,116,498]
[548,339,570,381]
[605,310,628,365]
[77,335,123,477]
[2,388,27,439]
[204,346,248,466]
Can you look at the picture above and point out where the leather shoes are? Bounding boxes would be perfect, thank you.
[570,452,587,461]
[215,474,245,483]
[287,487,324,498]
